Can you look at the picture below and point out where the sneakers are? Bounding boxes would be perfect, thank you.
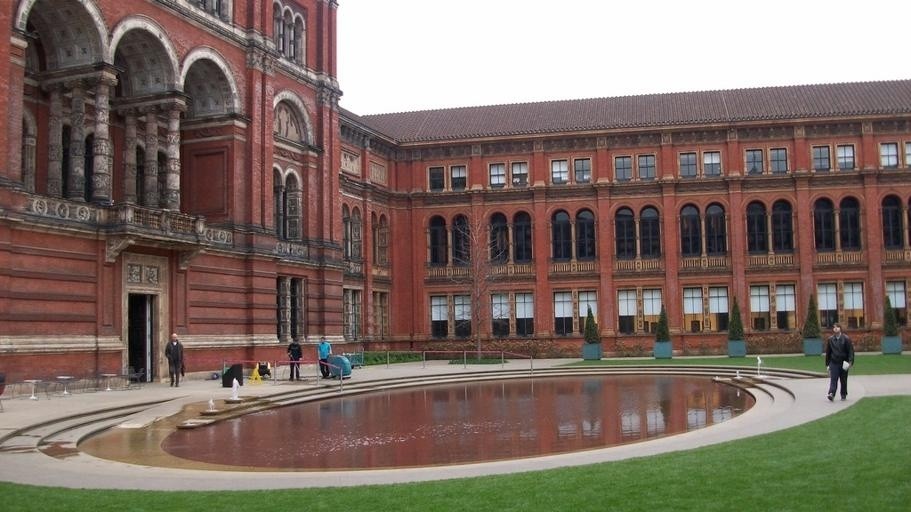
[827,393,834,402]
[841,394,846,400]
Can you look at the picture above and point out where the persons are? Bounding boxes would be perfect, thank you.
[825,323,854,401]
[165,332,184,387]
[288,338,302,382]
[318,337,332,379]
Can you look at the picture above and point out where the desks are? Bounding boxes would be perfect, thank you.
[25,372,118,401]
